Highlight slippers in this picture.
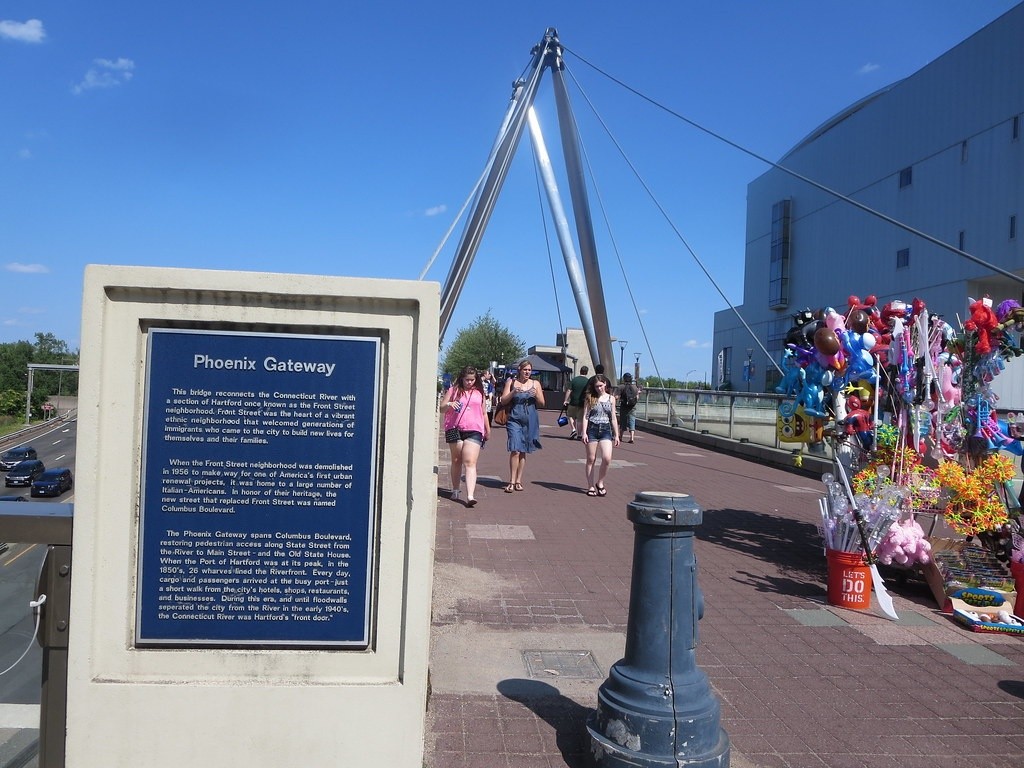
[586,486,597,497]
[596,484,607,496]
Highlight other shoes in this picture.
[450,489,461,500]
[467,497,477,507]
[577,435,582,441]
[619,436,623,442]
[627,441,634,443]
[567,430,577,439]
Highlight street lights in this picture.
[745,348,753,393]
[617,340,629,384]
[686,370,696,390]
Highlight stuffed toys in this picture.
[965,299,1003,354]
[872,519,931,568]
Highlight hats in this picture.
[623,373,633,377]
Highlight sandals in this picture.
[505,482,514,493]
[515,482,524,491]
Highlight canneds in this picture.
[454,401,463,413]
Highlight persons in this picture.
[563,364,611,440]
[615,373,639,443]
[582,375,620,497]
[479,370,517,426]
[1010,452,1024,626]
[440,366,491,506]
[502,361,545,493]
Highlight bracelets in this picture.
[448,402,450,407]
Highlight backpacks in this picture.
[622,382,637,406]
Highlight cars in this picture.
[30,467,72,498]
[6,459,45,488]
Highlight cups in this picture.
[454,402,463,413]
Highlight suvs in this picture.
[1,446,37,472]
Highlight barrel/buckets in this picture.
[824,543,875,610]
[1010,559,1024,619]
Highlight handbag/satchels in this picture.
[494,379,515,426]
[557,405,568,427]
[445,428,461,443]
